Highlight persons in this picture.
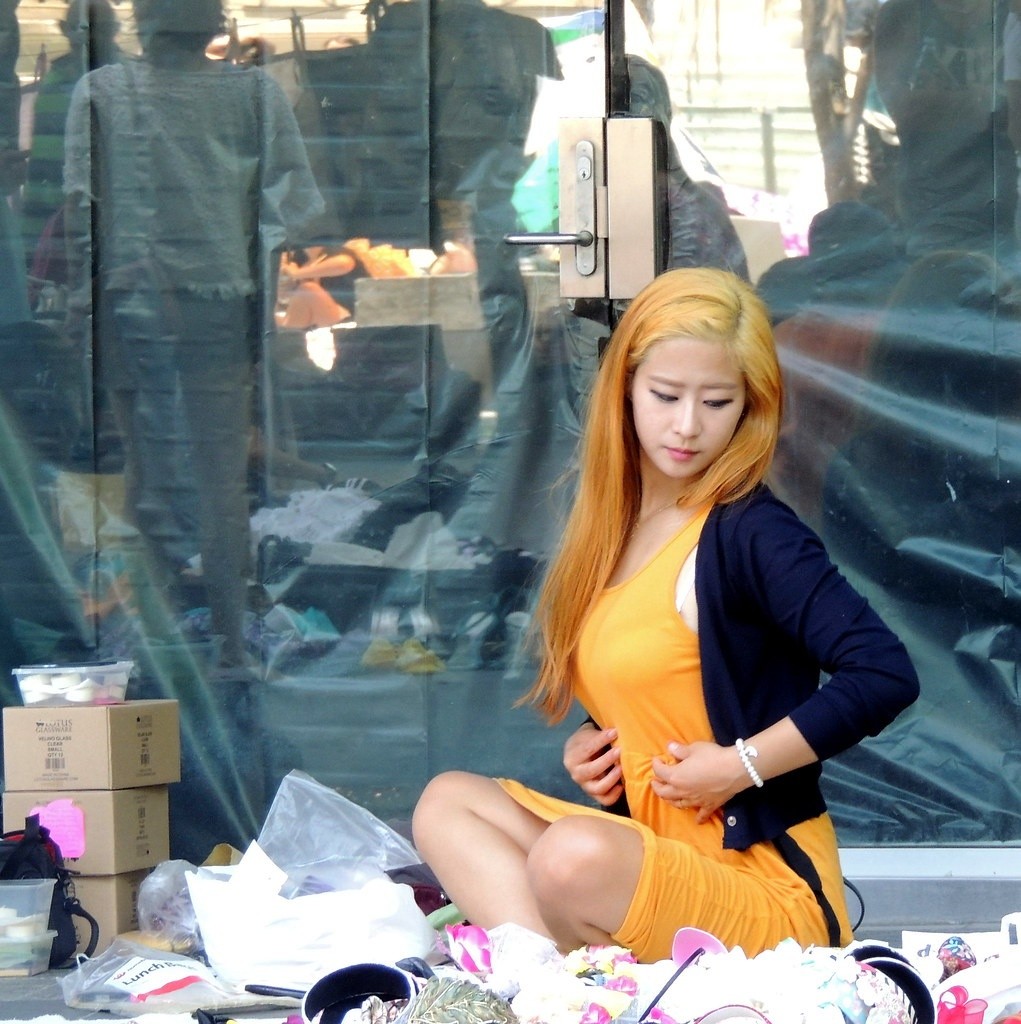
[0,0,1021,845]
[412,265,924,964]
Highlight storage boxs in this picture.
[355,274,574,413]
[0,659,180,978]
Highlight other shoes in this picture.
[504,614,541,673]
[447,614,504,669]
[361,637,399,669]
[397,638,448,675]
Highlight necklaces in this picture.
[622,500,677,543]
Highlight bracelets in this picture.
[734,738,764,788]
